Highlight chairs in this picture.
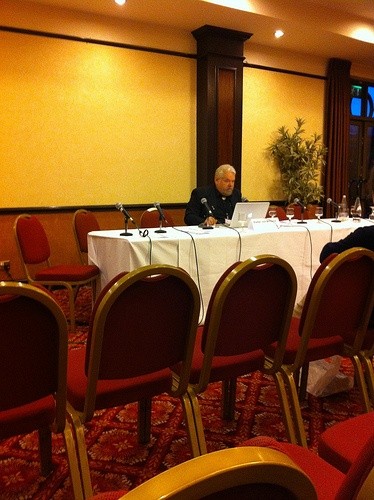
[0,201,374,500]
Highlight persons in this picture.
[296,225,374,311]
[184,164,242,225]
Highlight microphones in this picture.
[294,198,306,209]
[327,198,339,209]
[201,198,213,215]
[115,203,136,225]
[154,201,166,221]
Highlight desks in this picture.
[86,217,374,327]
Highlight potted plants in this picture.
[264,115,328,212]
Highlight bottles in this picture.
[354,197,361,218]
[338,194,349,221]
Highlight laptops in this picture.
[223,202,270,228]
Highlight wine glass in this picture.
[350,206,357,221]
[314,206,324,223]
[368,206,374,221]
[285,207,295,222]
[268,205,278,218]
